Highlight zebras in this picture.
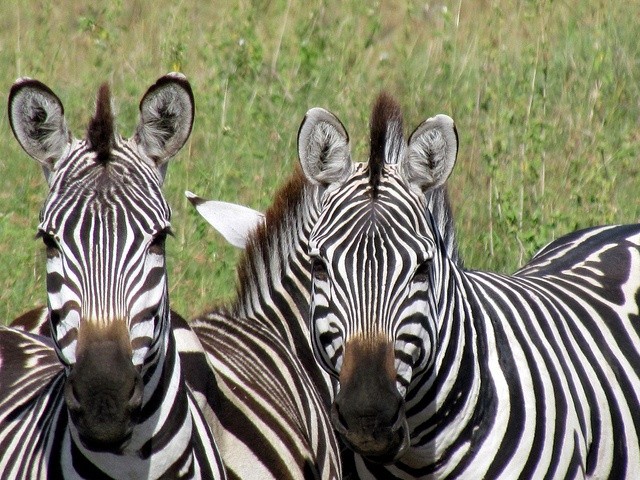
[295,89,639,480]
[183,166,341,480]
[1,72,227,480]
